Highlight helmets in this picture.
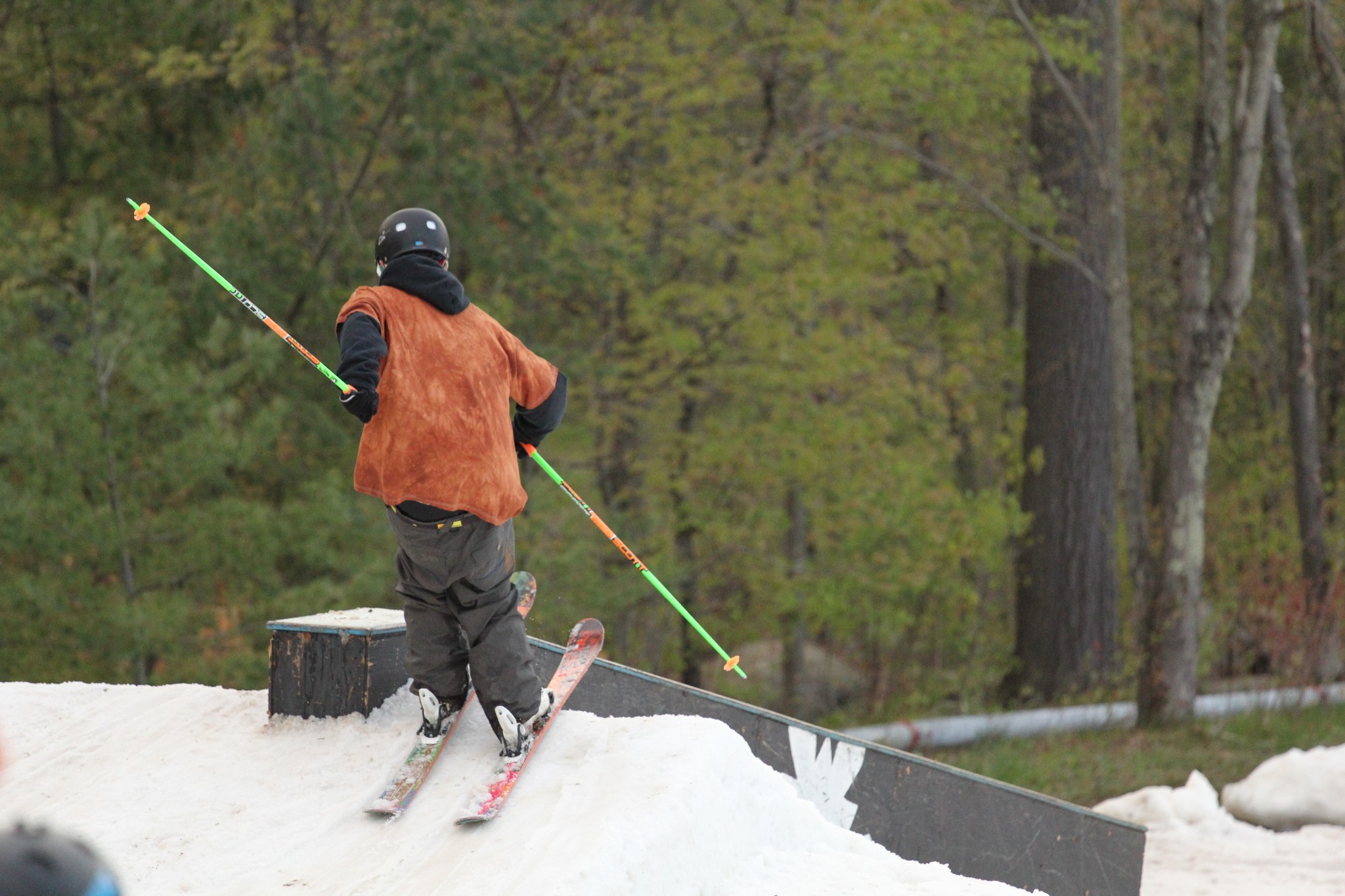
[373,207,448,265]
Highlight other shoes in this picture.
[419,687,460,744]
[493,688,554,771]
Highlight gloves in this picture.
[339,387,379,423]
[511,423,540,460]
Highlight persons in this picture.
[332,207,569,745]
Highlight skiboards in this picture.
[367,570,605,828]
